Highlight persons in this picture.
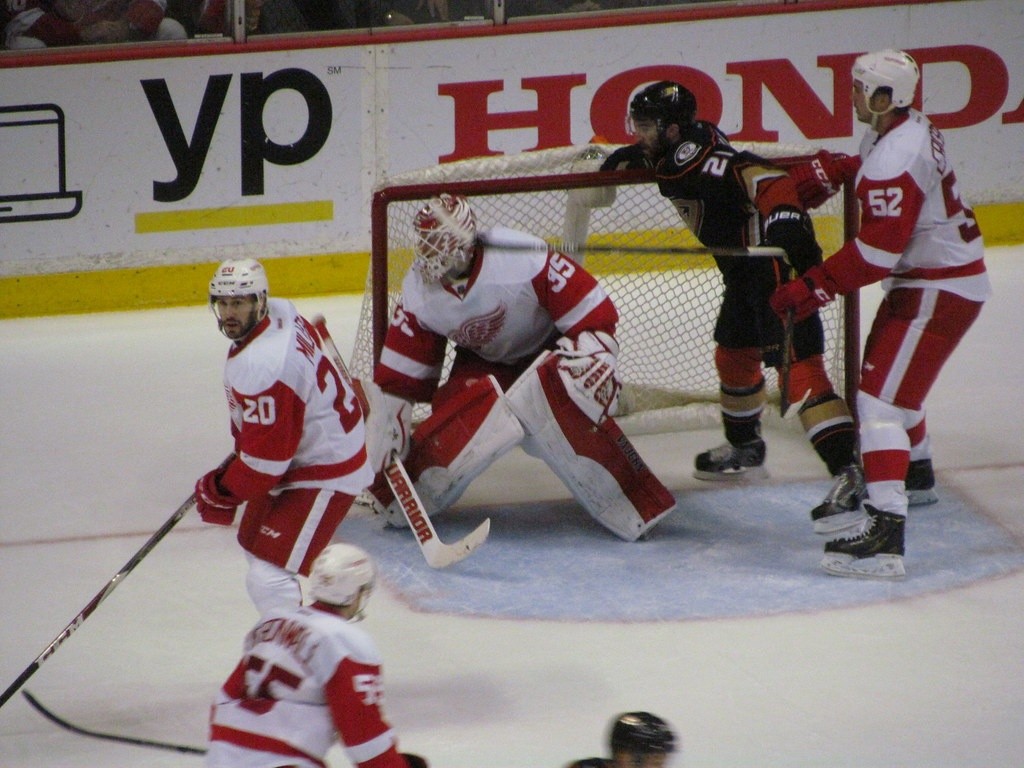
[364,194,680,541]
[565,713,677,768]
[772,49,997,582]
[601,81,871,533]
[193,257,375,617]
[204,543,427,768]
[0,0,719,51]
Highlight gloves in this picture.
[767,265,836,323]
[601,145,653,171]
[790,150,842,208]
[765,205,819,262]
[196,467,242,525]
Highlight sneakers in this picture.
[904,457,939,505]
[694,420,765,480]
[821,504,906,576]
[811,463,870,533]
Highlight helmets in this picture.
[414,194,477,284]
[307,543,373,606]
[628,80,694,140]
[611,712,675,754]
[209,257,268,320]
[854,47,920,114]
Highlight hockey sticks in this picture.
[310,313,491,569]
[21,690,208,754]
[429,196,785,257]
[780,264,813,420]
[0,492,197,708]
